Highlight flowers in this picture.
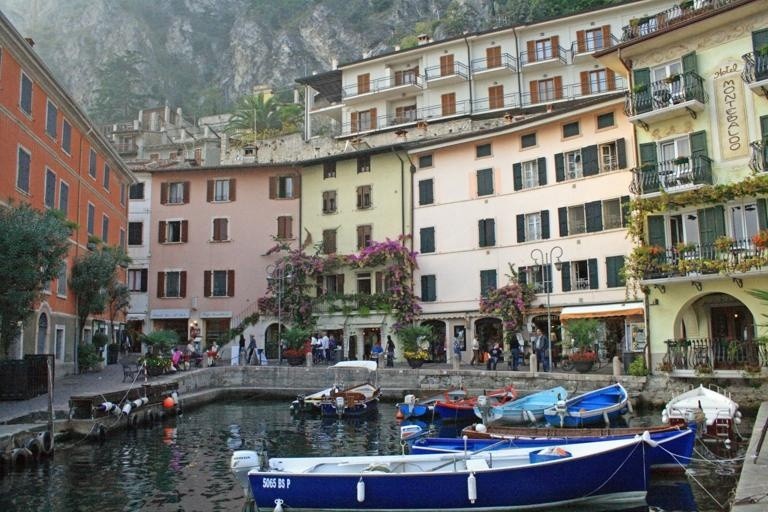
[568,351,596,363]
[283,348,307,360]
[403,349,431,361]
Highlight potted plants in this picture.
[93,356,106,372]
[138,356,166,377]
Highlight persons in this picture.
[171,329,556,374]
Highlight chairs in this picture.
[119,358,138,382]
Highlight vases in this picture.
[575,361,591,373]
[407,357,423,368]
[286,356,304,367]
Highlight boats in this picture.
[645,472,697,510]
[70,377,184,440]
[227,431,653,511]
[290,357,382,419]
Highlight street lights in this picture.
[529,244,566,371]
[265,261,292,366]
